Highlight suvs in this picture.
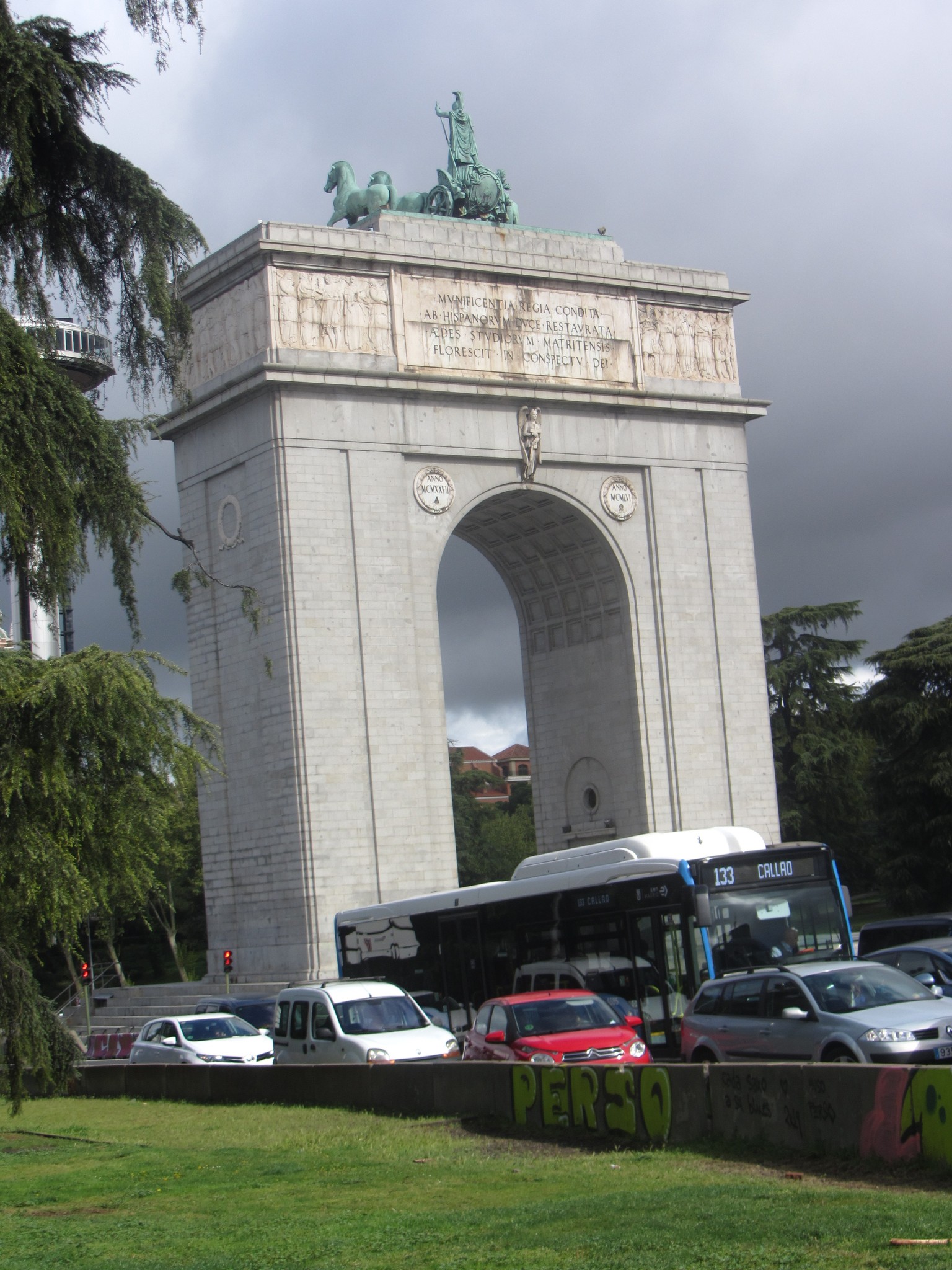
[678,955,952,1065]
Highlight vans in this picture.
[273,974,462,1066]
[511,954,692,1045]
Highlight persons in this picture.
[173,275,268,397]
[767,927,800,964]
[272,267,393,356]
[843,979,866,1008]
[516,402,542,482]
[435,91,484,187]
[638,303,738,384]
[204,1020,227,1040]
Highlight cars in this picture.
[128,1011,274,1065]
[861,935,952,994]
[461,988,654,1065]
[193,993,277,1038]
[406,989,479,1043]
[858,913,952,958]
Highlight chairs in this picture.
[774,982,821,1020]
[550,1004,579,1030]
[360,1006,387,1032]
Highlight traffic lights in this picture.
[224,950,233,972]
[80,961,90,984]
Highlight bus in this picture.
[333,826,857,1064]
